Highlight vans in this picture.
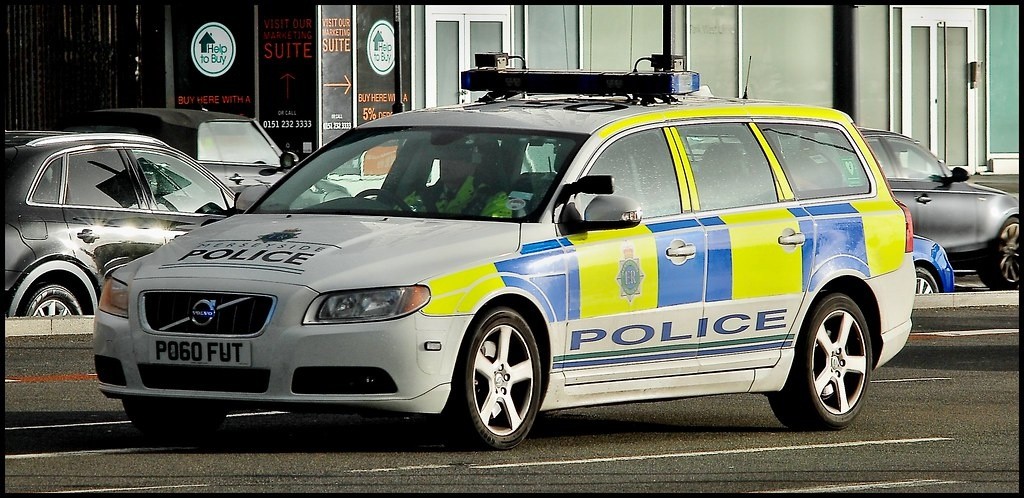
[94,108,289,185]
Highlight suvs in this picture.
[2,131,240,319]
[858,126,1022,292]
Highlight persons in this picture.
[393,139,512,218]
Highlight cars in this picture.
[91,59,915,450]
